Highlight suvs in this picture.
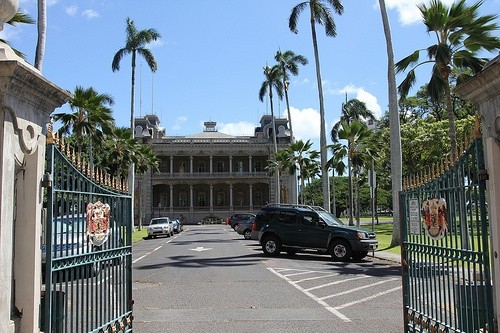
[227,213,255,229]
[41,212,125,277]
[250,203,379,262]
[146,216,174,239]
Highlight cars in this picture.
[236,215,256,240]
[171,220,183,234]
[230,216,253,231]
